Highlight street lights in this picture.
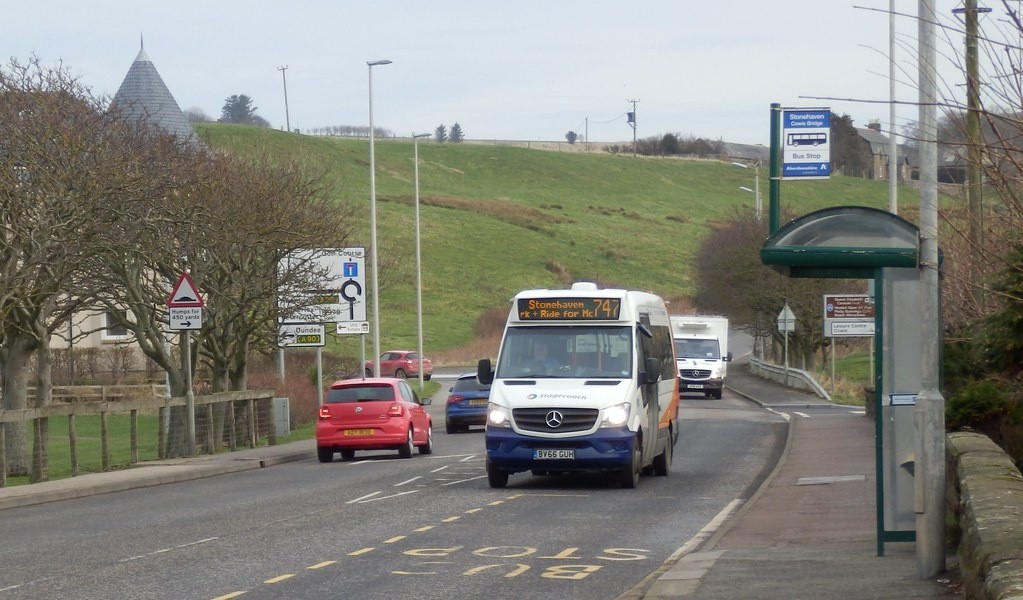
[365,60,396,378]
[410,132,433,391]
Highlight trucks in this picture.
[667,314,732,400]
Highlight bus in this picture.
[787,133,826,147]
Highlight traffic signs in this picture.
[337,321,369,334]
[275,324,325,348]
[169,307,202,330]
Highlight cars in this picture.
[444,371,495,434]
[316,377,433,458]
[365,350,433,382]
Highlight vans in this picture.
[476,282,680,490]
